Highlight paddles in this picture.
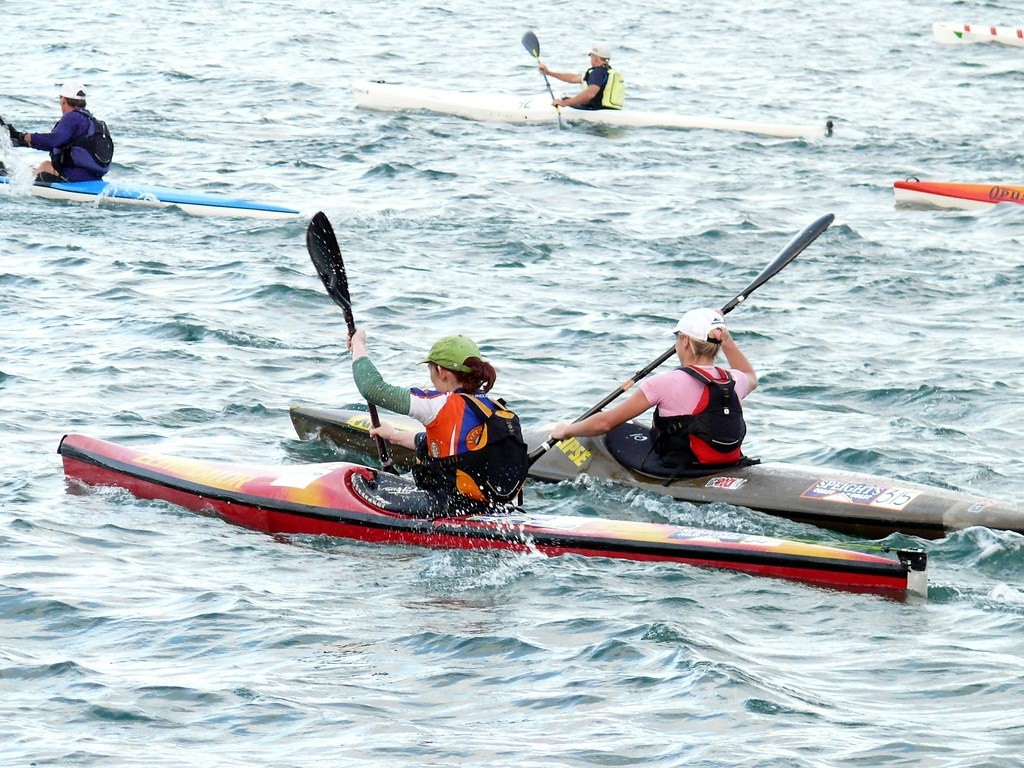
[520,32,572,131]
[304,208,403,474]
[530,213,840,467]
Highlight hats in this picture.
[54,81,87,100]
[663,308,725,344]
[417,335,481,373]
[588,47,610,58]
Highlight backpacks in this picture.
[73,109,113,168]
[597,65,625,110]
[456,393,529,506]
[674,367,747,464]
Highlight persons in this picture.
[538,47,612,110]
[551,308,757,467]
[347,326,495,504]
[4,83,104,181]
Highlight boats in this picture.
[896,175,1024,215]
[0,169,299,221]
[58,431,927,595]
[354,79,862,142]
[289,398,1024,540]
